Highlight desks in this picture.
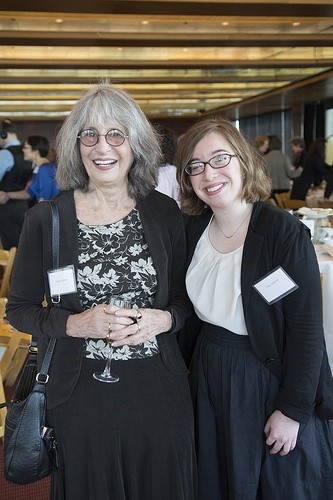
[309,227,333,371]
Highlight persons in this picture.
[251,134,310,212]
[0,120,61,249]
[173,118,333,500]
[153,127,183,209]
[5,78,192,499]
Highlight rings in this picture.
[103,305,106,313]
[106,333,109,340]
[136,323,141,333]
[108,324,110,331]
[136,308,143,321]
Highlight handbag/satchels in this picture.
[3,344,58,485]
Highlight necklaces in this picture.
[214,209,252,240]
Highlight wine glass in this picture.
[93,297,131,382]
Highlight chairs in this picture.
[268,190,333,222]
[0,247,16,299]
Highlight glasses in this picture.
[75,128,130,147]
[182,154,239,176]
[22,146,33,150]
